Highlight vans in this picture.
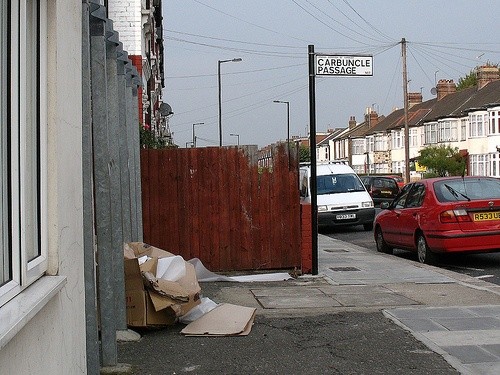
[299,162,375,230]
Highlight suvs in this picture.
[358,175,399,207]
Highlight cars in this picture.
[375,176,500,266]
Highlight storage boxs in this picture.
[121,240,204,330]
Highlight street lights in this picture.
[191,122,206,147]
[217,57,243,149]
[273,100,290,141]
[230,132,240,147]
